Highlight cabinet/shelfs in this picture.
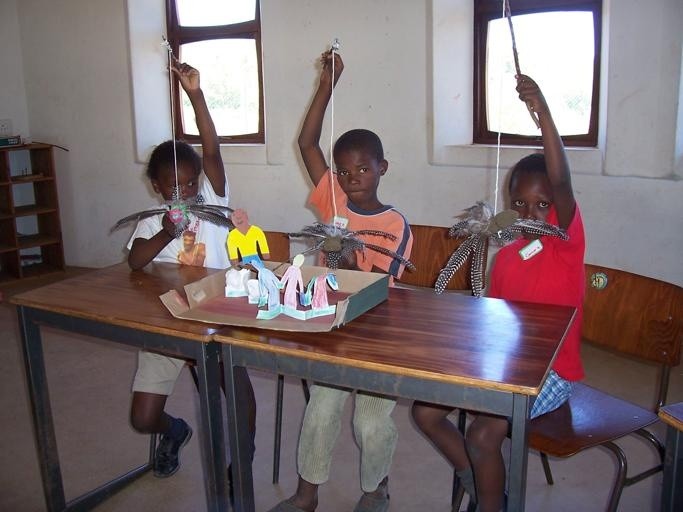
[0,143,67,304]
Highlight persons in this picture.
[115,51,226,478]
[421,69,587,511]
[267,52,415,512]
[175,229,206,267]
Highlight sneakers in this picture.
[153,418,193,479]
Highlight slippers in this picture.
[353,493,390,512]
[268,499,315,512]
